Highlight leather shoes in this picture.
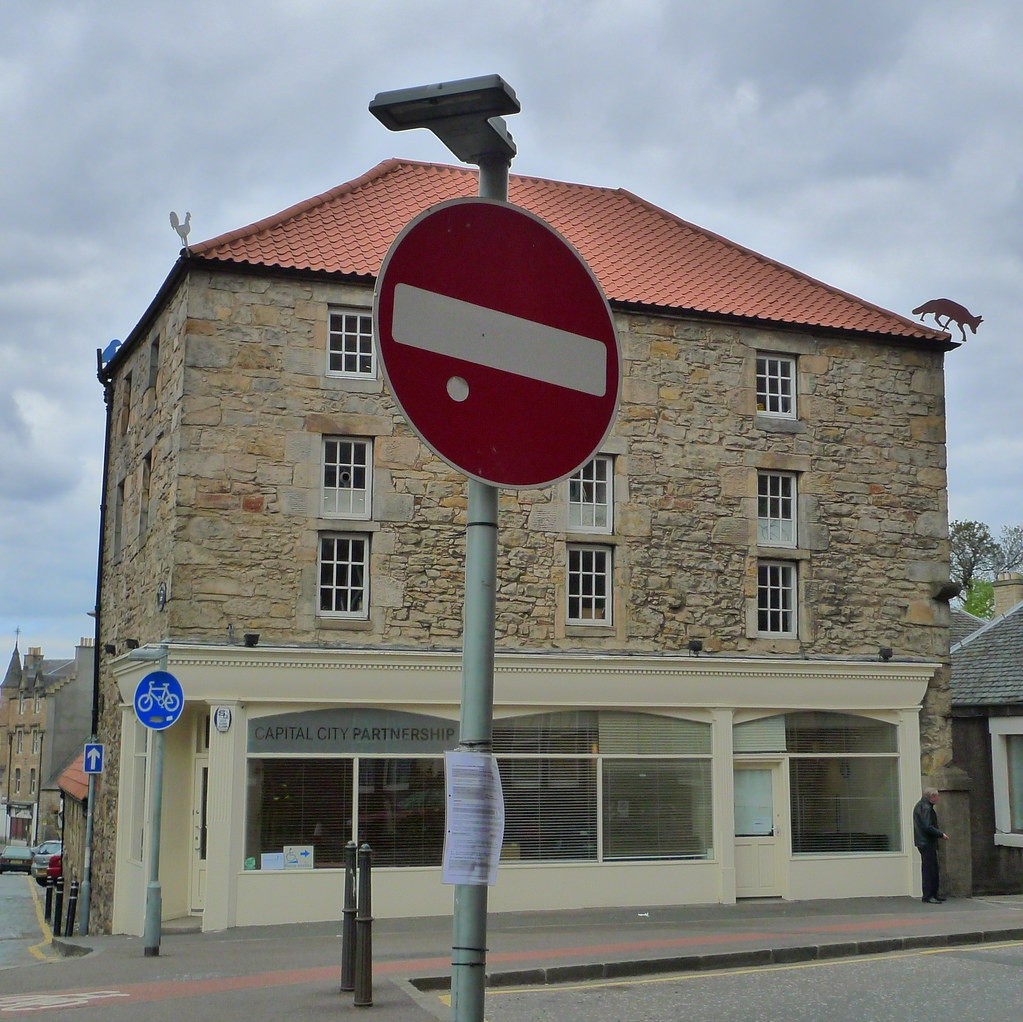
[928,897,941,904]
[935,895,946,901]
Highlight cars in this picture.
[0,846,35,875]
[31,840,63,887]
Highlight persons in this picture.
[912,786,949,903]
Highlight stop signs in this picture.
[370,195,622,494]
